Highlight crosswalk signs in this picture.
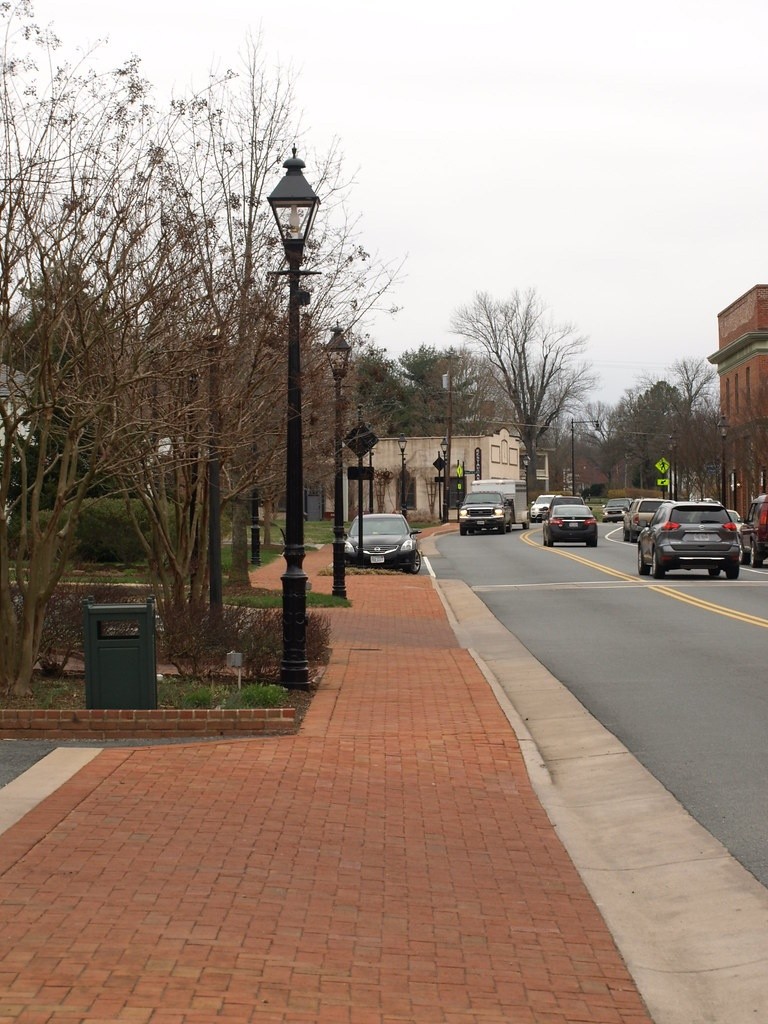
[655,458,670,474]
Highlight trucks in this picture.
[456,479,530,536]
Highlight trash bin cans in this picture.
[81,595,158,710]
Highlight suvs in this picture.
[531,495,593,523]
[737,495,768,568]
[622,498,676,544]
[637,500,744,580]
[602,498,633,523]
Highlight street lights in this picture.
[667,440,673,500]
[267,143,321,692]
[323,319,352,598]
[522,452,530,508]
[398,433,408,518]
[353,405,365,569]
[717,413,730,508]
[671,433,679,501]
[570,418,601,496]
[440,435,449,524]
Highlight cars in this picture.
[344,514,422,575]
[543,505,598,547]
